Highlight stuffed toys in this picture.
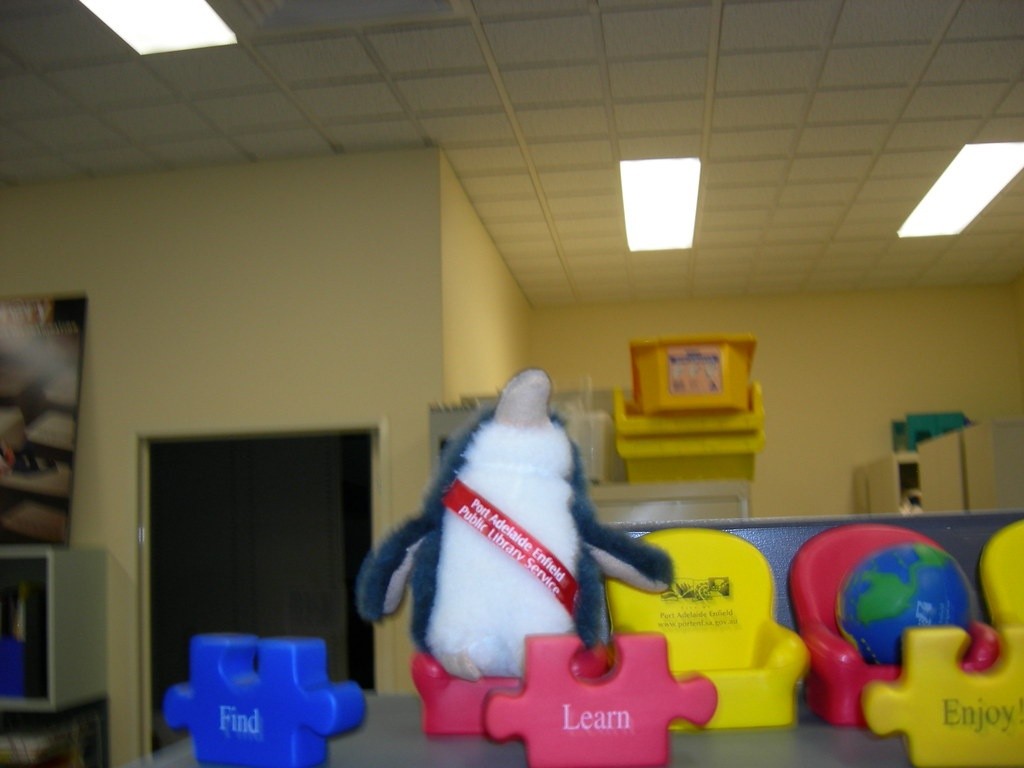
[356,367,673,682]
